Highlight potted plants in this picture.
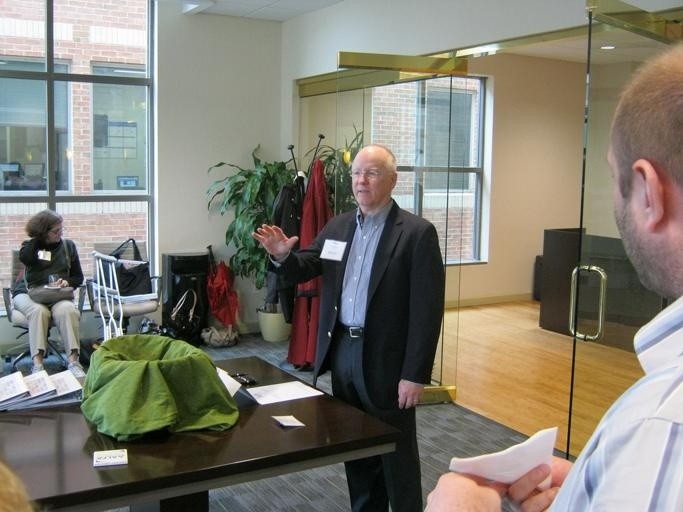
[205,141,307,343]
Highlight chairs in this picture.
[3,238,165,372]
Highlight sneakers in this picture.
[67,361,84,373]
[30,364,44,374]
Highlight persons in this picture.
[419,37,683,512]
[9,208,88,381]
[250,142,446,512]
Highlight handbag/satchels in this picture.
[135,315,167,336]
[28,281,75,304]
[85,236,142,312]
[201,324,239,347]
[78,333,113,365]
[162,288,201,349]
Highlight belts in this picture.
[335,321,363,338]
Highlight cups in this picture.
[48,274,59,287]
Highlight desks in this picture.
[0,354,400,511]
[539,226,672,356]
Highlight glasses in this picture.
[48,226,65,234]
[348,169,378,180]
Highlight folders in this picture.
[19,370,84,410]
[0,371,31,412]
[7,369,57,412]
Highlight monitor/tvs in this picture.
[0,162,21,184]
[116,176,140,187]
